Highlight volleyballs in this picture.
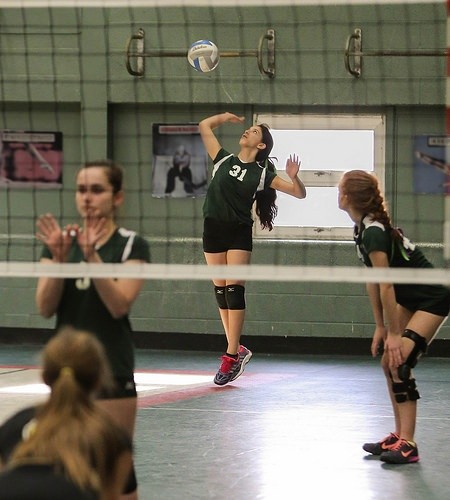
[188,39,220,73]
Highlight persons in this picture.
[34,160,152,500]
[198,111,307,386]
[0,326,134,500]
[338,171,450,464]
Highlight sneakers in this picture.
[363,433,400,455]
[231,345,253,381]
[214,352,239,385]
[380,439,419,463]
